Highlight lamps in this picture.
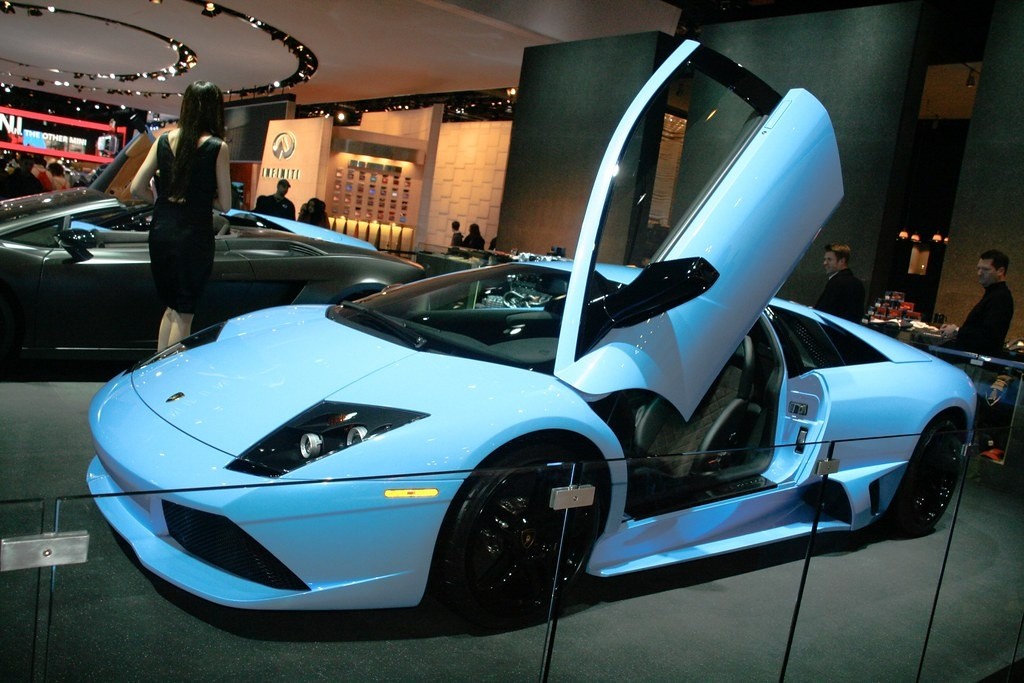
[201,2,222,17]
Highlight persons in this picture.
[940,249,1015,372]
[449,221,485,251]
[253,179,330,229]
[129,80,232,353]
[0,151,70,200]
[815,241,867,324]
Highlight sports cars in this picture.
[82,30,978,636]
[0,126,432,381]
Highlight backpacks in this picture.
[33,165,52,192]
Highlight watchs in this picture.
[953,326,959,336]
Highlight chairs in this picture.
[635,334,756,508]
[213,215,230,237]
